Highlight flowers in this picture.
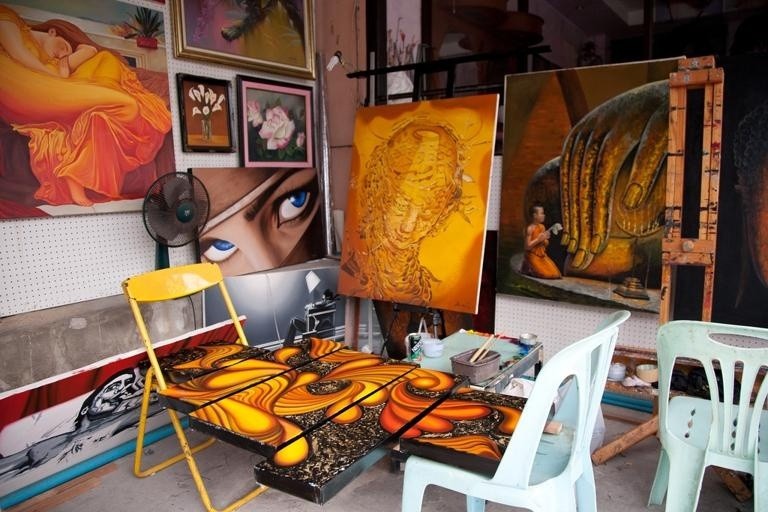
[246,93,308,161]
[185,82,226,120]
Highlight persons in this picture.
[559,78,670,273]
[282,289,334,347]
[341,117,477,306]
[0,368,169,476]
[519,204,562,279]
[1,6,139,207]
[193,167,323,278]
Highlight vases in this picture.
[199,118,213,143]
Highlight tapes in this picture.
[636,364,658,382]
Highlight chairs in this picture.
[645,318,768,511]
[302,304,337,342]
[119,261,271,512]
[398,309,634,512]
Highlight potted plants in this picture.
[121,6,167,53]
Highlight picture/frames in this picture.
[170,0,319,84]
[173,69,239,158]
[233,73,317,169]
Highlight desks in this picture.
[401,327,544,396]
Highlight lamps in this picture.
[304,270,322,294]
[327,52,354,75]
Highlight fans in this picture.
[138,168,213,300]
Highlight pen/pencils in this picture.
[469,334,501,364]
